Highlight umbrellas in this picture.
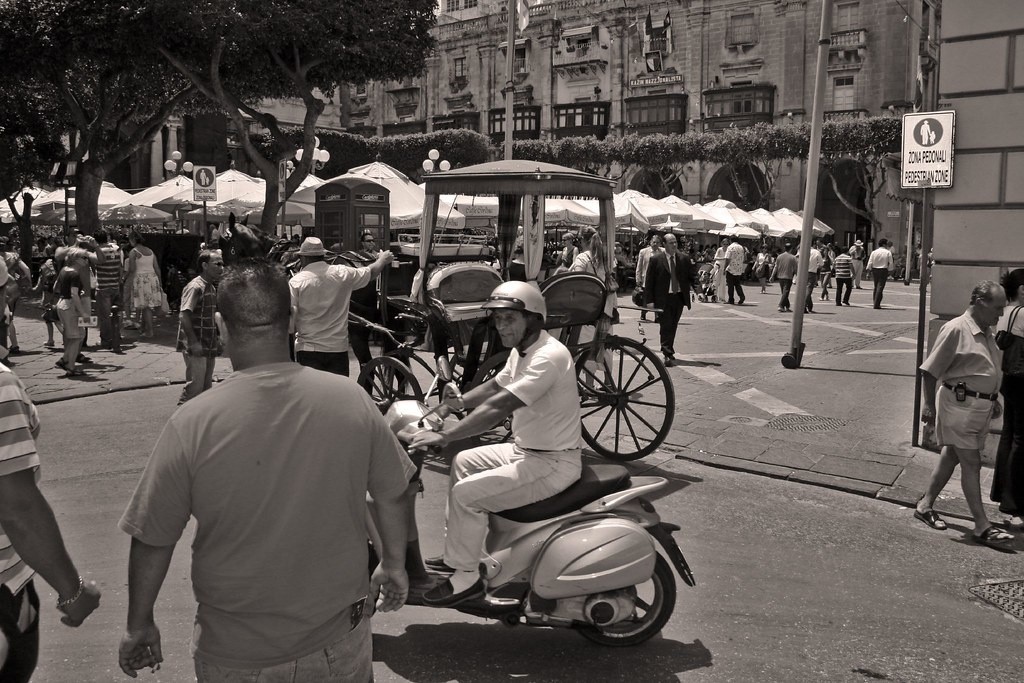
[0,156,844,258]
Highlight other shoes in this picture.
[425,555,456,572]
[1,326,158,377]
[422,573,484,607]
[664,353,674,366]
[717,289,881,314]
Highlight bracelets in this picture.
[56,576,84,607]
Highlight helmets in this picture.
[481,281,546,327]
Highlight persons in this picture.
[912,279,1016,543]
[989,269,1024,517]
[558,233,581,268]
[833,247,856,305]
[695,237,751,304]
[866,239,894,309]
[634,236,665,323]
[0,222,397,403]
[570,225,620,401]
[805,242,825,314]
[116,255,420,683]
[888,241,896,254]
[0,251,104,683]
[406,279,584,608]
[644,232,704,360]
[771,244,798,312]
[849,239,866,289]
[752,238,836,301]
[509,234,528,284]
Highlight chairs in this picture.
[427,262,506,322]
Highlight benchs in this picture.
[539,273,619,329]
[398,234,494,256]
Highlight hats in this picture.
[854,240,864,246]
[294,236,327,256]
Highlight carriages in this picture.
[217,160,675,463]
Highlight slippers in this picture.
[971,525,1014,544]
[913,509,949,530]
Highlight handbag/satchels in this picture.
[632,286,645,306]
[605,274,619,293]
[995,305,1024,374]
[156,288,169,312]
[48,274,57,293]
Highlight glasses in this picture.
[364,239,375,242]
[208,261,226,268]
[80,256,90,260]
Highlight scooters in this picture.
[366,354,696,650]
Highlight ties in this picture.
[670,256,678,295]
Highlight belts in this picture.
[522,447,579,453]
[943,381,999,402]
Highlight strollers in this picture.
[691,263,721,303]
